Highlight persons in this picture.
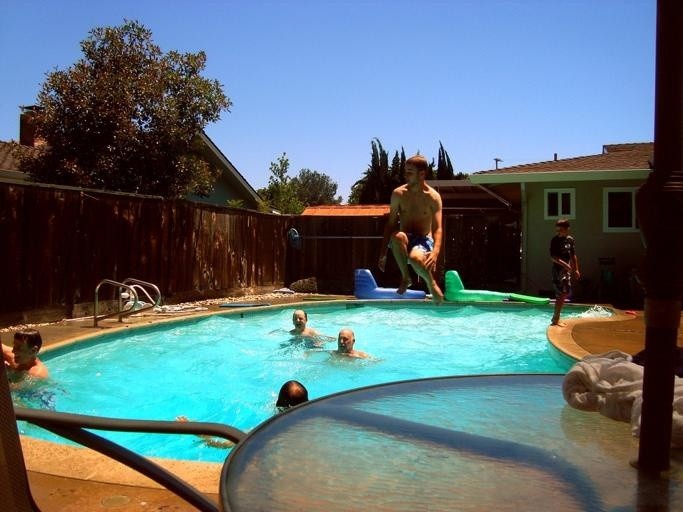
[177,380,308,450]
[549,217,582,327]
[323,329,376,363]
[1,328,57,416]
[376,155,449,302]
[289,306,321,338]
